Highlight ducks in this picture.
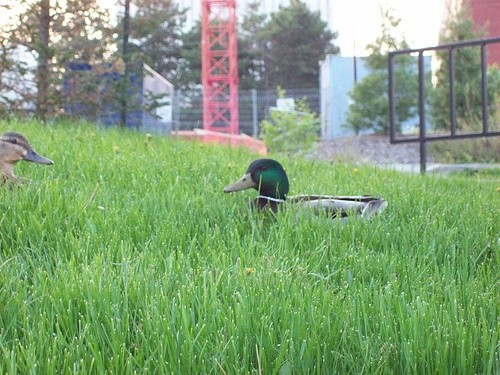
[224,158,388,220]
[0,130,53,188]
